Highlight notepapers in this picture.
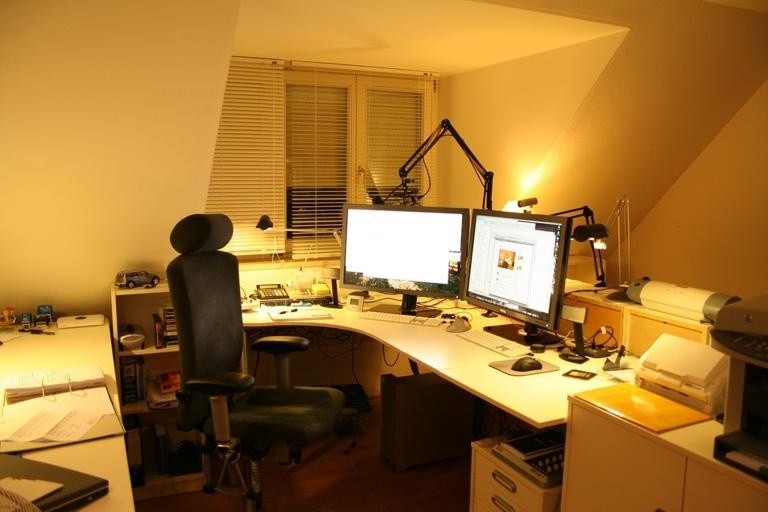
[311,283,330,295]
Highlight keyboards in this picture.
[457,329,531,359]
[359,311,443,327]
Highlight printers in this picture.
[706,290,767,485]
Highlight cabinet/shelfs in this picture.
[560,386,768,512]
[108,280,230,500]
[464,434,563,511]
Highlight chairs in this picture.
[166,213,359,507]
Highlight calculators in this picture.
[256,284,289,298]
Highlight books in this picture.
[254,303,332,321]
[0,363,126,455]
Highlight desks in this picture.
[241,281,640,512]
[0,312,137,512]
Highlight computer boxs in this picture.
[380,373,478,472]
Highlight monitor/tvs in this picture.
[463,208,573,347]
[338,202,470,310]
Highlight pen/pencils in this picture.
[19,329,55,335]
[280,309,297,314]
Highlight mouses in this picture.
[511,357,542,371]
